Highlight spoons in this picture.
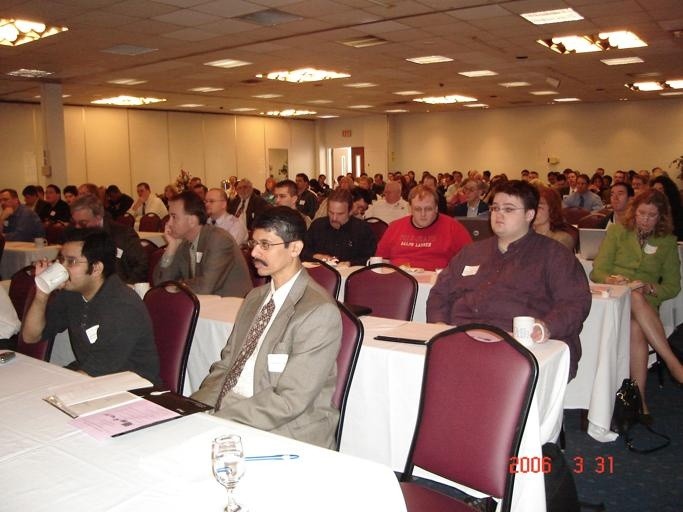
[244,452,303,463]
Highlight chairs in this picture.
[115,211,169,287]
[299,257,342,300]
[143,282,200,395]
[241,243,269,286]
[45,218,89,244]
[330,301,365,452]
[397,320,539,512]
[363,215,387,242]
[7,264,55,362]
[344,263,418,321]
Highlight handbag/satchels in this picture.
[611,378,641,435]
[543,443,578,511]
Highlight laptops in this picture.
[579,229,607,260]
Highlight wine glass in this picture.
[210,434,244,512]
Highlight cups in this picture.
[33,238,47,249]
[511,315,544,350]
[365,256,382,272]
[33,262,70,294]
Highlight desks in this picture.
[49,282,571,511]
[317,254,632,444]
[0,241,62,280]
[1,346,408,511]
[576,239,682,340]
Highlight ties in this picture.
[235,199,247,218]
[214,295,274,414]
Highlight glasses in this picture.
[248,240,293,249]
[489,204,525,214]
[55,256,88,267]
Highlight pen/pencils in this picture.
[245,454,300,460]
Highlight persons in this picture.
[2,164,683,452]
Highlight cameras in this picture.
[0,352,15,363]
